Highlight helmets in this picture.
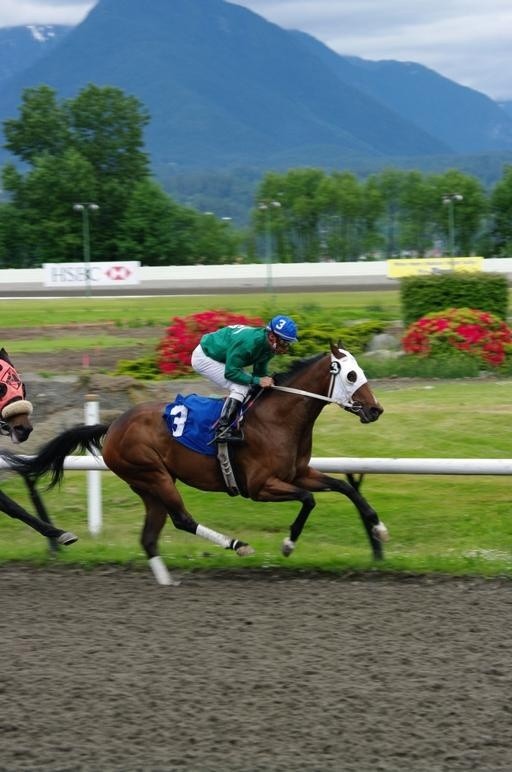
[266,314,297,341]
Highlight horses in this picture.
[1,343,79,547]
[14,336,392,588]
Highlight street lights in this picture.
[442,192,464,255]
[71,201,100,295]
[258,200,281,298]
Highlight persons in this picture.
[188,313,301,442]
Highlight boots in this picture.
[215,397,243,442]
